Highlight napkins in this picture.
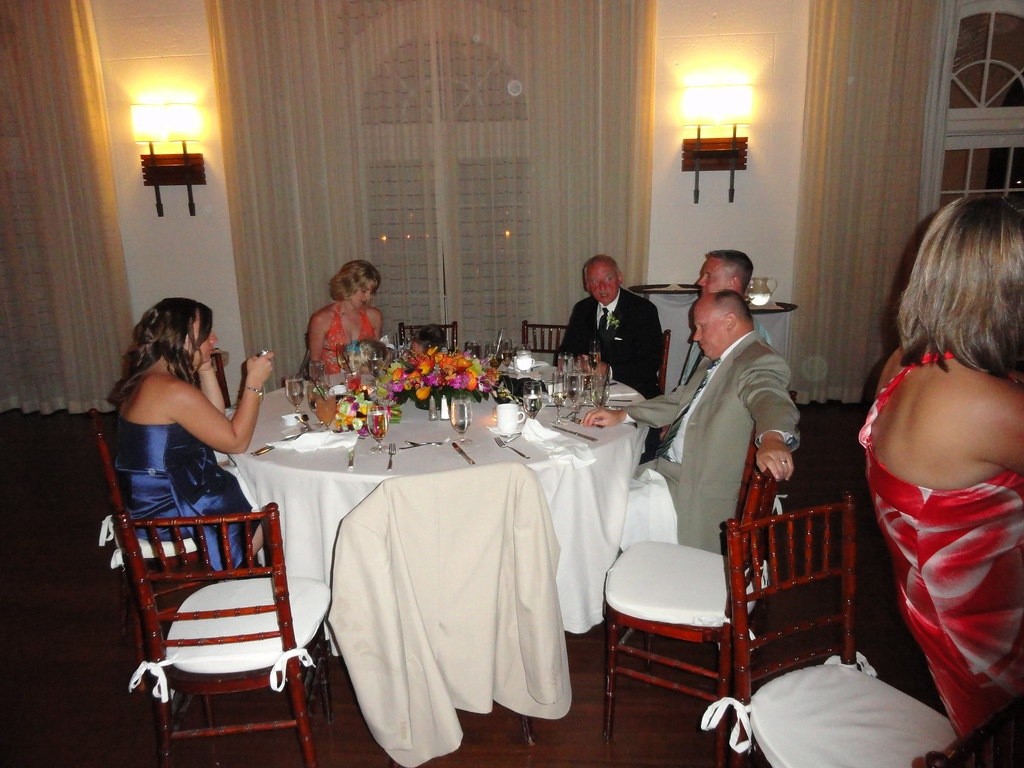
[270,429,359,452]
[521,418,562,445]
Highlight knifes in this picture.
[553,425,598,442]
[452,443,474,465]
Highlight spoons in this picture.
[405,440,441,445]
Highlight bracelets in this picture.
[245,385,262,396]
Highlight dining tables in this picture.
[216,356,648,768]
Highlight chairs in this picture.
[602,457,780,768]
[397,320,672,399]
[726,489,970,768]
[91,408,221,691]
[735,391,797,526]
[173,346,233,410]
[117,502,335,768]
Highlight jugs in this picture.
[745,277,777,306]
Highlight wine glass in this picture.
[285,339,610,454]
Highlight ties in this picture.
[655,359,721,459]
[598,307,609,347]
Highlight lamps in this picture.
[134,91,208,217]
[679,68,757,202]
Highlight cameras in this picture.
[493,375,548,403]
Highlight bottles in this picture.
[441,395,450,420]
[429,395,438,420]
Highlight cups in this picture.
[495,404,525,434]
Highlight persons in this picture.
[587,289,801,555]
[859,195,1024,736]
[308,260,392,375]
[407,324,446,355]
[111,298,275,571]
[554,254,664,400]
[681,249,771,386]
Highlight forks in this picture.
[493,437,530,459]
[399,437,450,450]
[387,443,396,469]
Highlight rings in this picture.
[781,461,787,464]
